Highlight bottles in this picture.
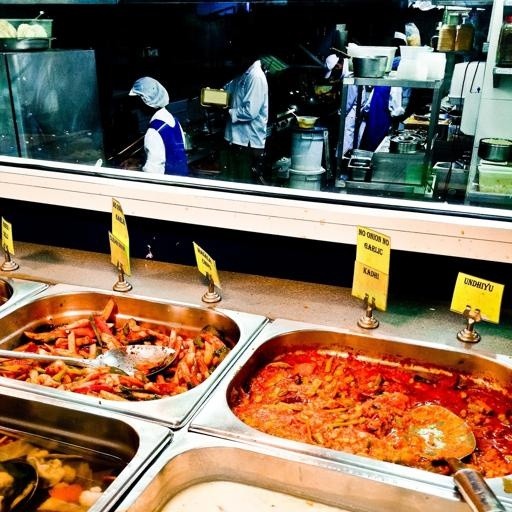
[495,14,512,68]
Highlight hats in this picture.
[322,54,340,79]
[126,75,171,109]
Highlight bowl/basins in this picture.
[296,115,321,128]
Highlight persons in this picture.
[320,52,356,83]
[205,35,271,186]
[336,68,366,173]
[120,74,189,181]
[354,56,412,156]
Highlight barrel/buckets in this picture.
[292,132,323,171]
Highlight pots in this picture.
[478,138,512,162]
[388,132,427,154]
[330,47,388,78]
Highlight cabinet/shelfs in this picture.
[335,72,443,196]
[465,1,512,209]
